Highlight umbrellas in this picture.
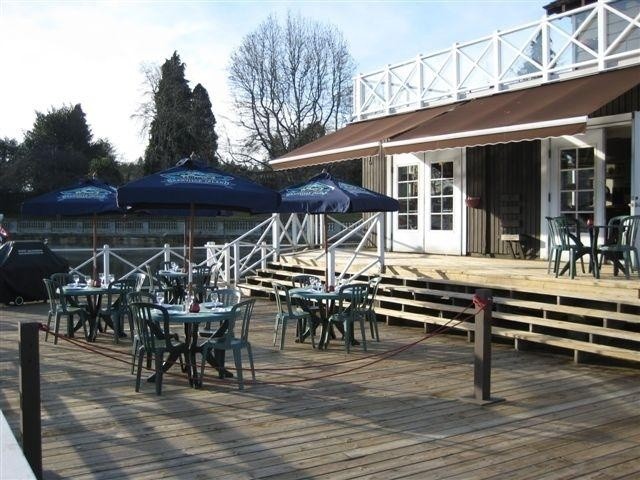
[118,152,281,342]
[19,176,124,283]
[278,167,399,317]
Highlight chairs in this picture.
[545,215,639,280]
[273,275,382,354]
[42,273,146,344]
[156,261,217,305]
[125,290,258,395]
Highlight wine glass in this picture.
[314,279,322,293]
[156,292,167,306]
[108,274,114,286]
[171,261,177,271]
[99,273,104,285]
[309,276,315,291]
[210,293,218,310]
[84,275,91,288]
[73,274,80,286]
